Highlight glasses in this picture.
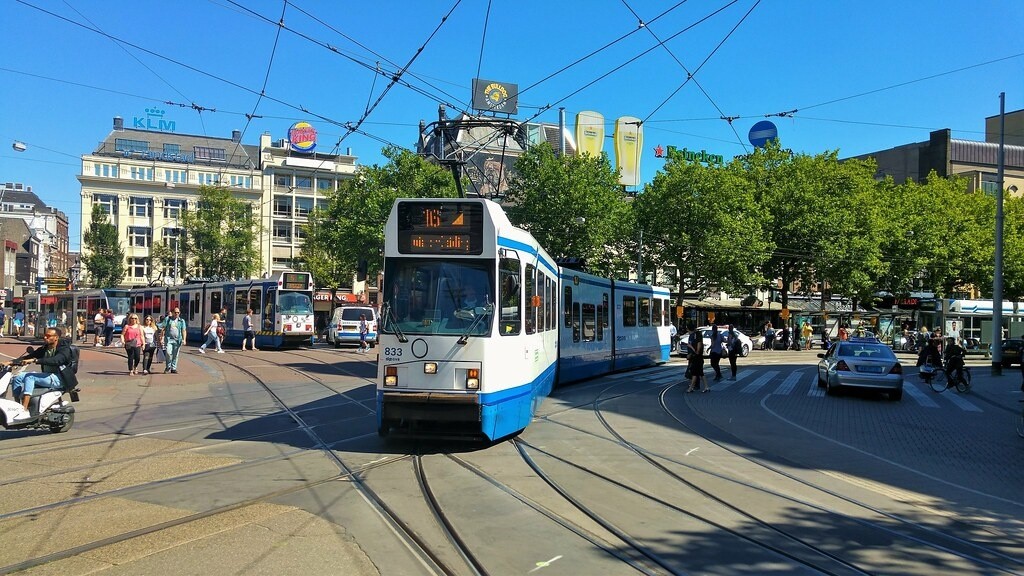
[131,312,180,321]
[45,334,57,338]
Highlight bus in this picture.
[22,288,132,337]
[11,296,26,336]
[919,298,1024,350]
[128,270,318,349]
[375,105,682,441]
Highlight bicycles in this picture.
[929,355,972,394]
[1014,396,1024,439]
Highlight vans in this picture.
[324,306,379,349]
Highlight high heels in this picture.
[702,388,710,393]
[687,387,695,392]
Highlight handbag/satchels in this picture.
[126,338,137,349]
[106,318,115,327]
[157,347,165,361]
[720,337,742,357]
[685,367,692,380]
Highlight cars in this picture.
[675,327,753,359]
[1002,337,1024,366]
[817,335,904,401]
[750,327,792,351]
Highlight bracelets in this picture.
[33,358,37,362]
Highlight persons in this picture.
[670,320,968,395]
[0,301,329,420]
[948,322,961,347]
[446,280,488,330]
[355,314,371,353]
[624,311,657,326]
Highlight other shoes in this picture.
[714,374,722,380]
[356,347,362,351]
[171,370,179,373]
[964,384,971,393]
[365,347,370,352]
[252,348,259,351]
[144,370,148,374]
[14,410,31,419]
[198,348,205,353]
[164,368,171,374]
[217,350,225,353]
[129,368,138,375]
[242,349,247,351]
[0,334,3,338]
[93,343,110,347]
[727,377,736,380]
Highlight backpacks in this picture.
[205,324,212,332]
[68,345,79,373]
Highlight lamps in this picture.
[163,182,175,190]
[12,142,26,152]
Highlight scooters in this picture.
[0,346,82,435]
[918,364,938,382]
